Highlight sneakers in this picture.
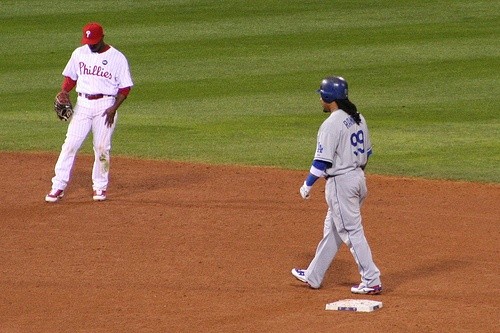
[92,189,107,201]
[291,268,320,289]
[351,284,382,295]
[45,188,65,202]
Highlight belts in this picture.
[79,92,111,100]
[325,174,335,180]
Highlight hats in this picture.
[81,22,103,44]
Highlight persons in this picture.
[290,75,383,295]
[46,23,134,203]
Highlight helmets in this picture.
[317,76,348,103]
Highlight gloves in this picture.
[300,181,312,199]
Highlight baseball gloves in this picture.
[53,91,74,121]
